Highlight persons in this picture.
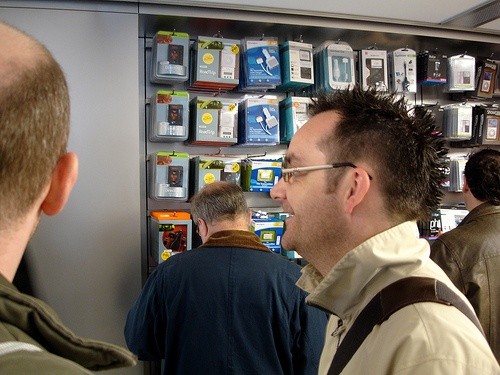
[123,181,328,375]
[431,149,500,363]
[269,83,500,375]
[0,22,137,375]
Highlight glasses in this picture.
[279,158,373,182]
